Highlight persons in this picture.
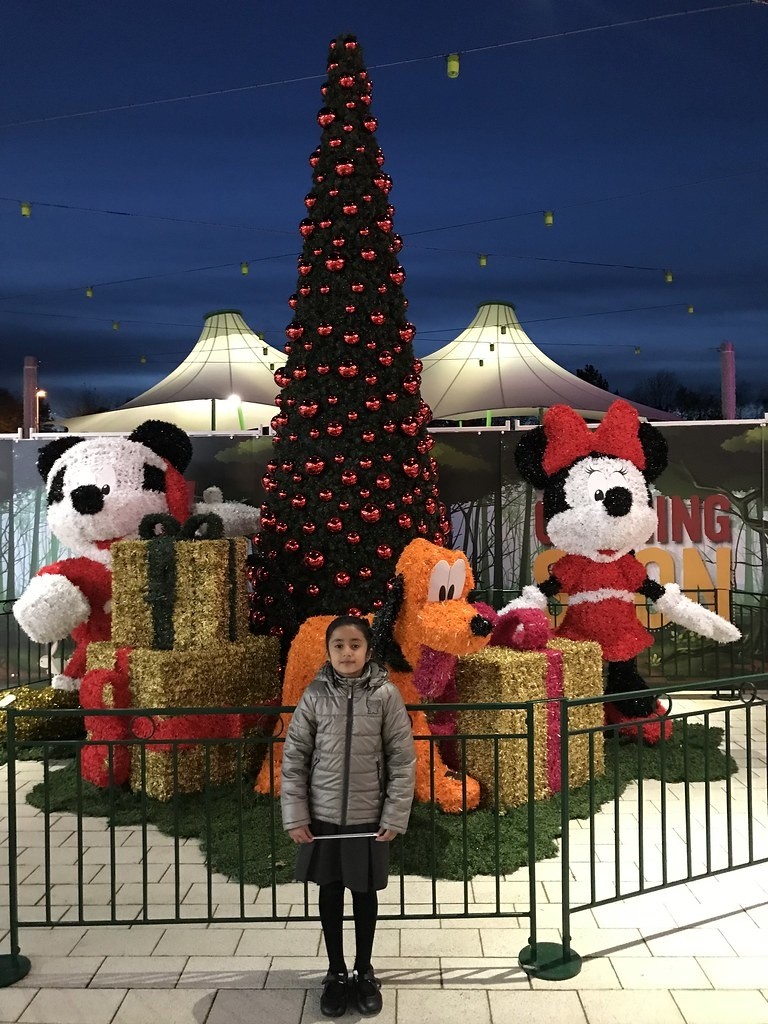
[279,616,415,1017]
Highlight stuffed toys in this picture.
[11,420,261,691]
[496,399,742,741]
[254,538,494,812]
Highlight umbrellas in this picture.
[419,303,680,426]
[47,309,288,432]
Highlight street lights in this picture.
[36,389,47,437]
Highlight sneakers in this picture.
[351,965,383,1015]
[320,964,350,1018]
